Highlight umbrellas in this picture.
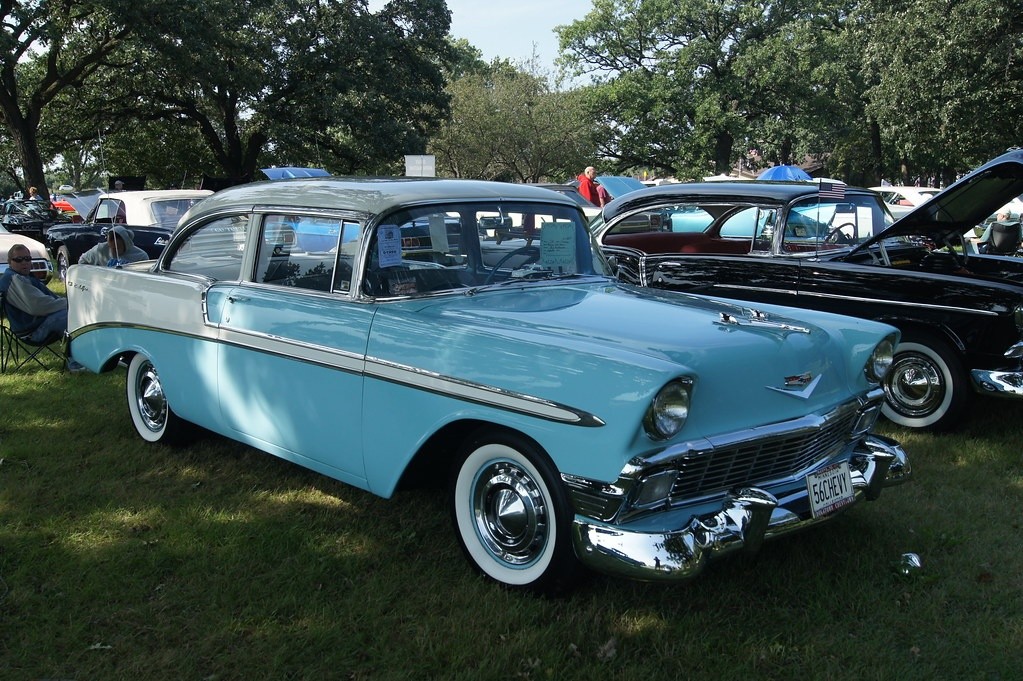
[757,163,816,182]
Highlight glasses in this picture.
[9,255,32,263]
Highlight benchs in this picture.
[274,268,478,306]
[172,256,354,289]
[603,230,720,267]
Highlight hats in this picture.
[115,181,124,185]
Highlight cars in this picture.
[47,188,249,280]
[446,183,601,237]
[0,200,61,243]
[260,167,461,253]
[481,149,1023,430]
[51,198,77,213]
[59,185,73,190]
[14,191,43,201]
[67,176,912,598]
[868,186,942,218]
[0,224,54,286]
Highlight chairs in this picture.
[0,296,71,372]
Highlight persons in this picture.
[49,192,66,202]
[576,164,602,208]
[115,180,125,191]
[79,225,149,268]
[596,183,610,208]
[28,186,43,201]
[1,194,15,203]
[1,242,88,371]
